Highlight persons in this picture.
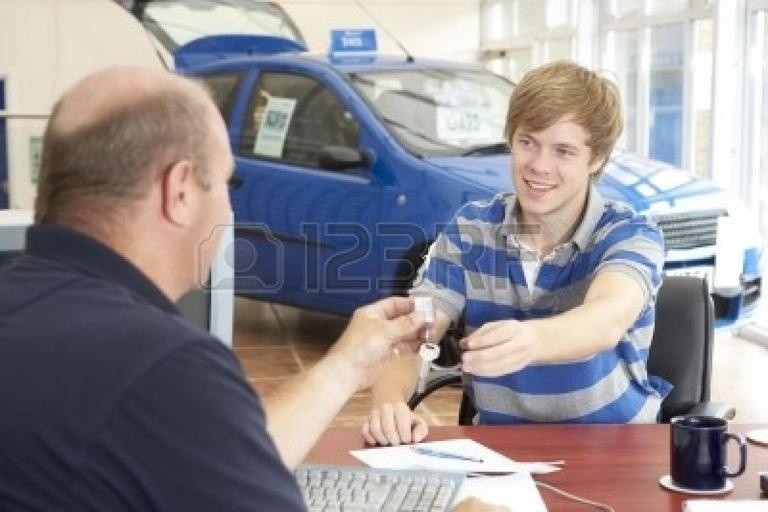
[362,59,674,447]
[0,64,511,512]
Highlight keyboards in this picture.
[285,467,464,512]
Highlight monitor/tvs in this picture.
[0,209,235,351]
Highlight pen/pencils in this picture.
[413,448,483,463]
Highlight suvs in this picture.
[113,1,762,370]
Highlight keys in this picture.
[417,342,441,395]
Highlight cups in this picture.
[670,414,748,491]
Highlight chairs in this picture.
[405,275,738,425]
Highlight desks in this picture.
[305,424,768,512]
